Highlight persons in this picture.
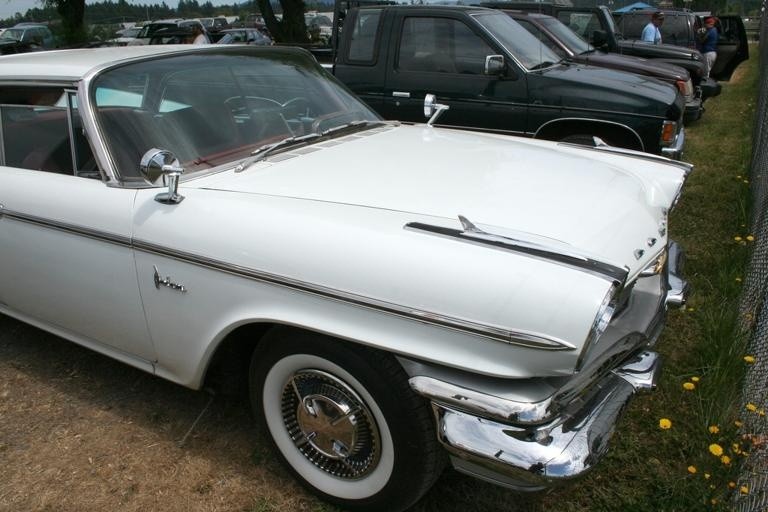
[190,22,207,44]
[309,24,328,47]
[641,11,664,44]
[696,18,719,79]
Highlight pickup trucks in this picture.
[296,2,687,165]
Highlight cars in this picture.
[1,24,55,55]
[106,10,338,46]
[488,0,760,127]
[0,40,696,510]
